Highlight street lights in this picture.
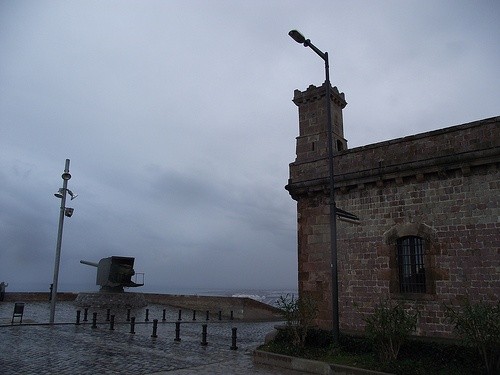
[49,158,79,324]
[289,30,359,347]
